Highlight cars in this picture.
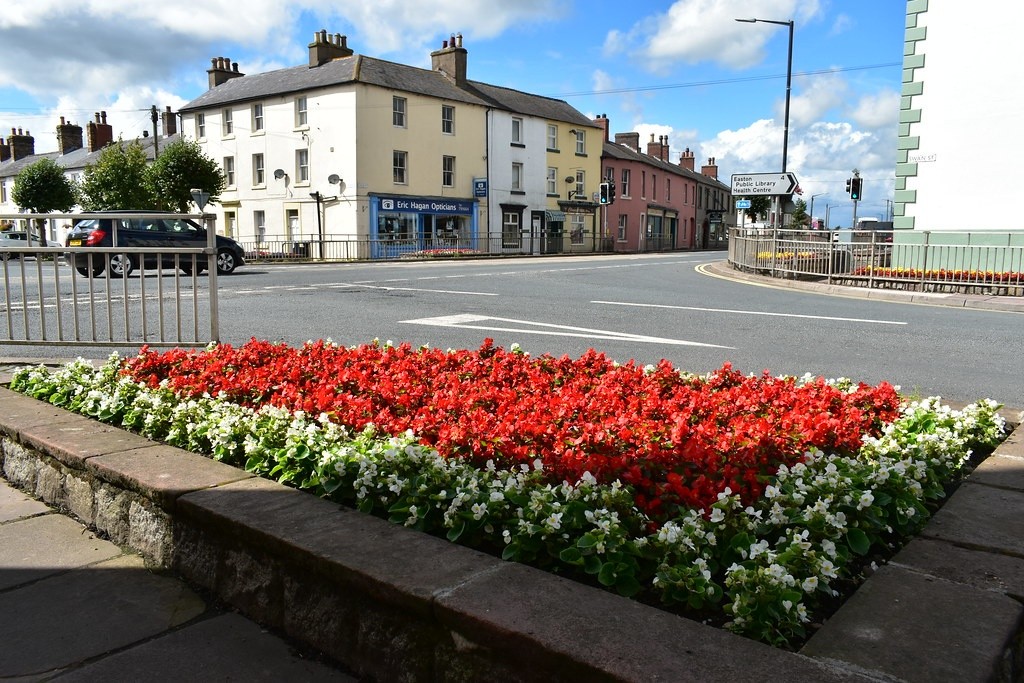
[0,231,63,261]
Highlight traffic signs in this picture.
[730,172,798,196]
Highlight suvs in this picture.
[63,207,245,279]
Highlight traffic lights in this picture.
[599,183,610,205]
[846,178,851,194]
[850,178,861,201]
[609,182,616,204]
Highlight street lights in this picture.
[733,17,795,172]
